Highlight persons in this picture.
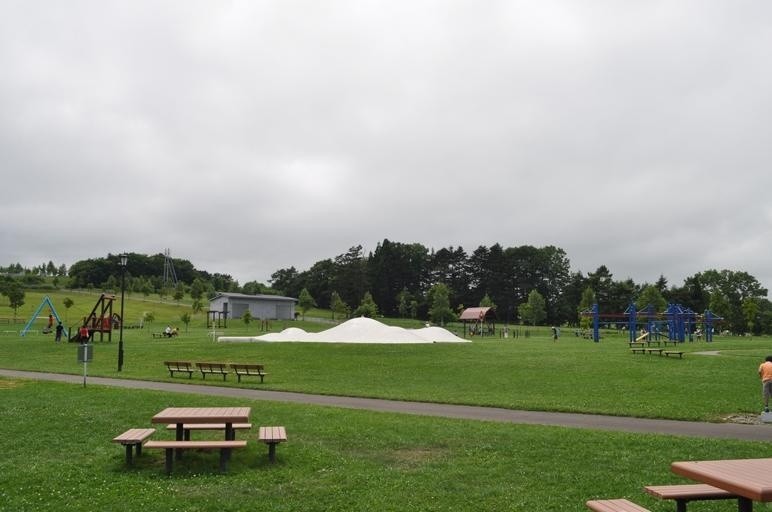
[166,325,172,338]
[171,326,180,338]
[47,314,55,328]
[640,327,646,342]
[79,325,90,344]
[54,322,63,341]
[92,311,98,328]
[757,354,772,412]
[552,326,560,342]
[695,326,702,343]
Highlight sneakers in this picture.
[765,407,769,412]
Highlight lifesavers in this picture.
[479,310,484,322]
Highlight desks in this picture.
[670,458,771,512]
[153,406,252,459]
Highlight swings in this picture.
[42,301,54,333]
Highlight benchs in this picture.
[642,484,743,512]
[163,361,268,383]
[111,429,156,466]
[166,422,253,441]
[259,424,287,462]
[586,497,651,512]
[145,440,247,475]
[628,341,686,358]
